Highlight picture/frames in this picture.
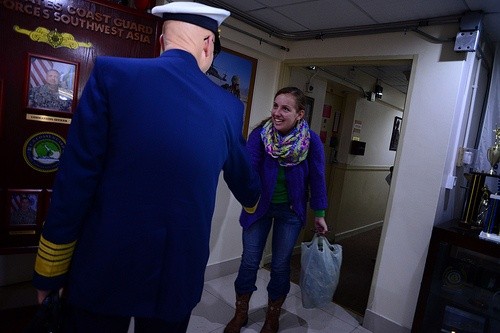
[6,189,52,232]
[206,46,258,141]
[22,53,79,117]
[390,117,402,151]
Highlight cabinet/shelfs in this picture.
[411,227,500,333]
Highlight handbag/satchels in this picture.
[300,233,342,308]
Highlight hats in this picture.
[152,2,231,54]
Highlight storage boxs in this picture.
[443,306,486,333]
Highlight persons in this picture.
[224,87,328,332]
[34,1,262,333]
[29,69,71,113]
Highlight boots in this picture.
[224,291,253,333]
[260,295,286,333]
